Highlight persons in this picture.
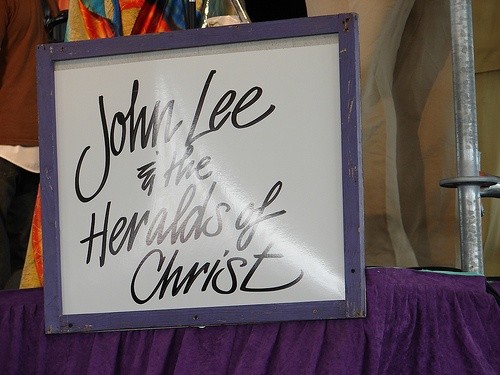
[0,0,57,294]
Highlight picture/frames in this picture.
[35,12,367,336]
